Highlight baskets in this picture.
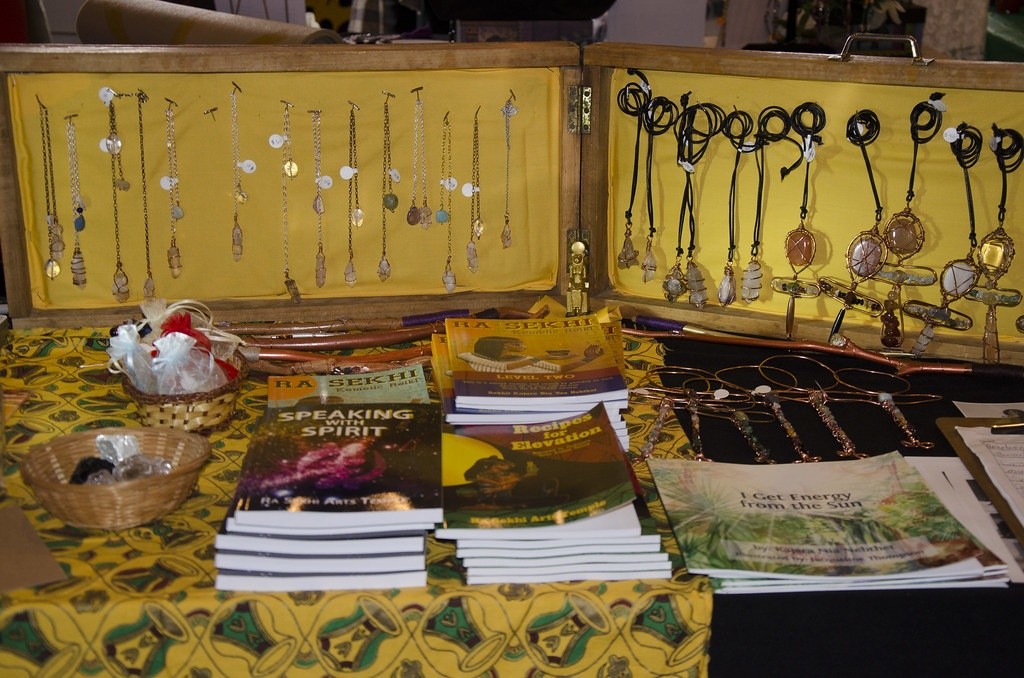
[18,425,213,532]
[121,352,249,433]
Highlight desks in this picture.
[0,306,1024,678]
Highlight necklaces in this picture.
[611,66,1024,365]
[466,116,484,273]
[502,101,518,250]
[165,108,183,281]
[376,103,398,283]
[406,101,432,229]
[436,118,456,292]
[67,116,85,290]
[229,89,246,262]
[99,91,155,304]
[282,106,303,306]
[346,110,364,288]
[310,113,326,289]
[37,103,64,283]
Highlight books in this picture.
[209,314,1017,596]
